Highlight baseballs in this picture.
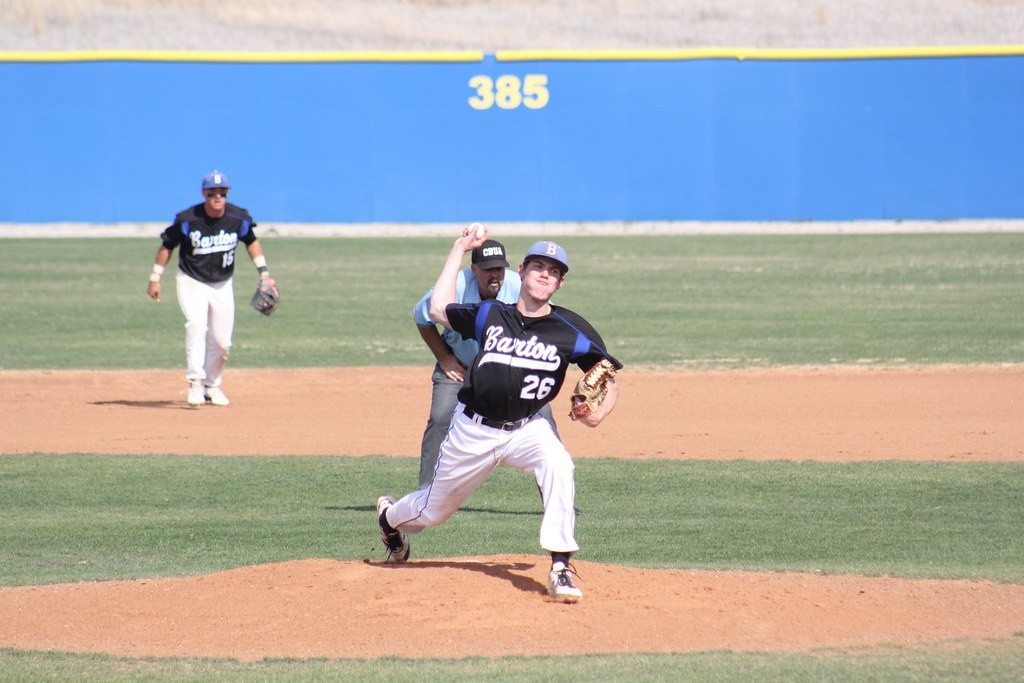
[468,222,485,239]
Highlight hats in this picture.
[202,170,230,189]
[523,240,569,273]
[472,240,510,269]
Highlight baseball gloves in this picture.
[567,357,620,428]
[249,278,280,316]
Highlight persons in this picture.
[376,222,623,603]
[146,170,280,406]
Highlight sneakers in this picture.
[376,494,411,564]
[547,561,586,603]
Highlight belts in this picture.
[463,406,534,430]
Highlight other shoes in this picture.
[205,387,230,406]
[186,380,206,405]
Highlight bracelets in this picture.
[149,264,164,282]
[253,255,269,276]
